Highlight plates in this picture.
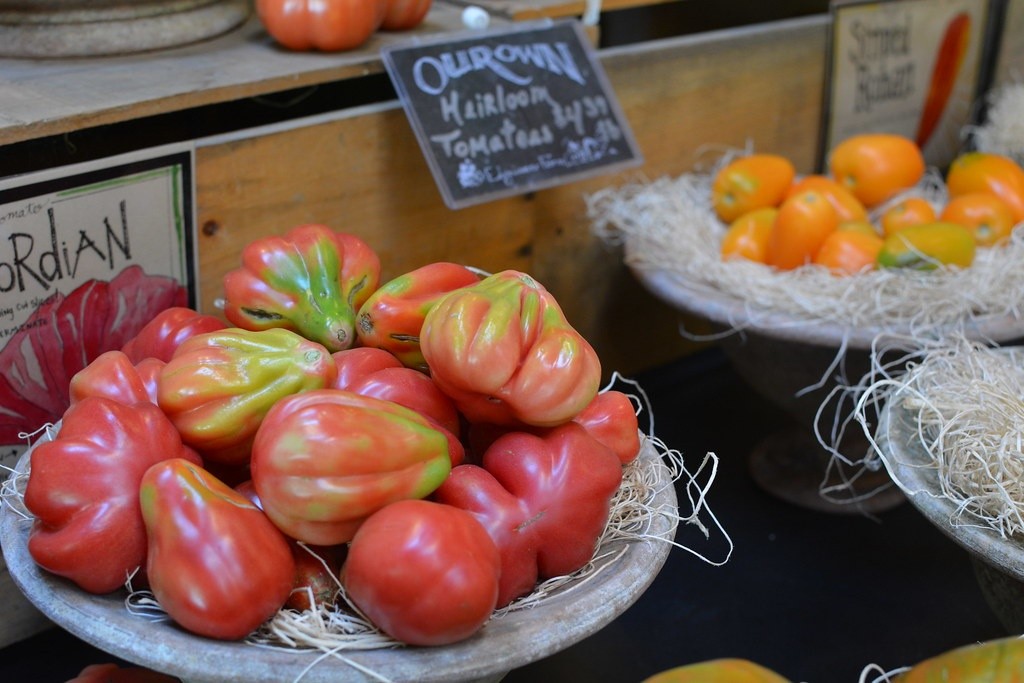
[0,419,678,683]
[621,236,1024,349]
[881,348,1024,576]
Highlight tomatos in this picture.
[710,135,1024,278]
[254,0,432,51]
[24,223,640,650]
